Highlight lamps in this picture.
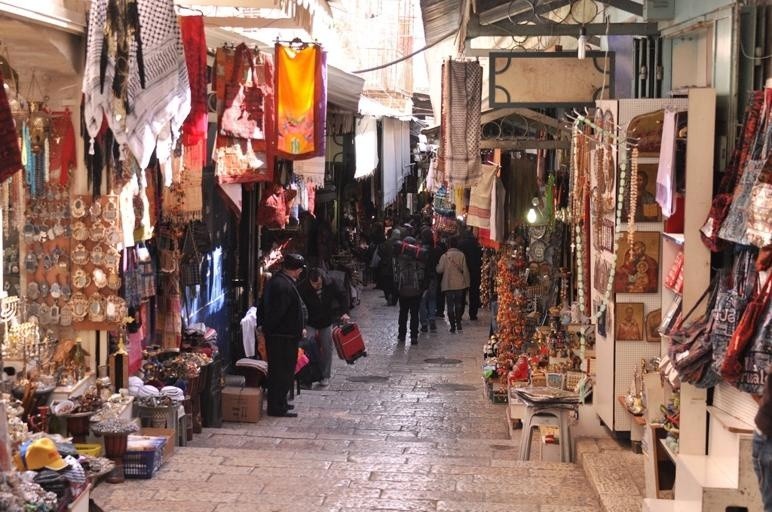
[526,158,537,224]
[578,0,586,60]
[532,184,540,206]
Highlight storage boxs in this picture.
[133,428,175,457]
[220,386,264,423]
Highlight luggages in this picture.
[330,319,368,364]
[298,338,325,386]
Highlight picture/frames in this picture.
[652,425,679,499]
[614,303,644,341]
[616,164,663,222]
[613,231,660,294]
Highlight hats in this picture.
[285,253,308,269]
[24,437,72,471]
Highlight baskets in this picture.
[123,438,167,480]
[74,442,103,457]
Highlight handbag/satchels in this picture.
[670,82,771,404]
[182,264,201,286]
[121,242,156,334]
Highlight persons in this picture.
[366,203,483,346]
[615,240,659,294]
[256,253,309,419]
[623,170,660,221]
[616,305,640,340]
[294,267,348,390]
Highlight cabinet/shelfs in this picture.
[661,88,714,499]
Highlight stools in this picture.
[133,397,180,446]
[518,406,573,462]
